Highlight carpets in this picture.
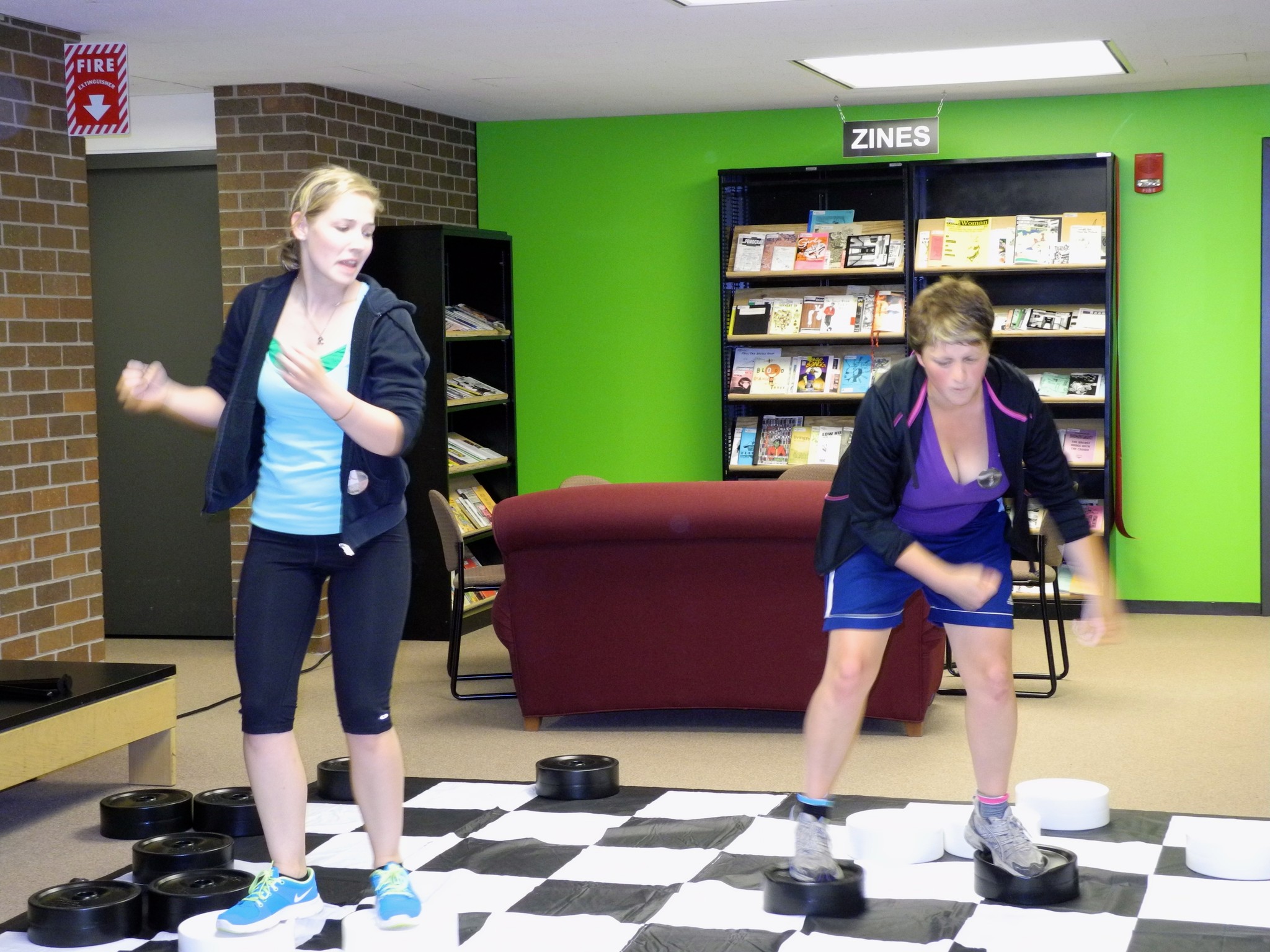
[0,773,1270,952]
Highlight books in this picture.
[448,437,505,466]
[729,210,1105,597]
[450,557,496,609]
[449,486,497,534]
[443,302,502,331]
[446,372,504,399]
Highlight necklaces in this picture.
[300,290,345,344]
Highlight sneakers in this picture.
[788,807,843,883]
[370,862,421,930]
[962,795,1045,879]
[217,860,323,934]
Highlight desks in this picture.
[0,660,179,790]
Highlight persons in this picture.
[115,164,430,934]
[790,279,1118,883]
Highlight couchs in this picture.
[492,482,946,736]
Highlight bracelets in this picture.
[332,397,356,421]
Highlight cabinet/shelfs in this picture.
[370,224,519,641]
[718,161,911,481]
[909,152,1119,620]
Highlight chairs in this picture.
[428,490,516,700]
[936,482,1069,698]
